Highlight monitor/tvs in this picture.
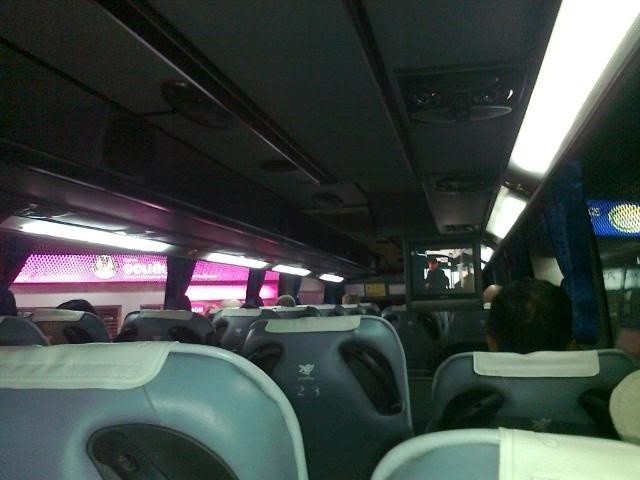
[404,233,484,314]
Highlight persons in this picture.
[486,277,573,354]
[424,257,449,291]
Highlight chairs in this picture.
[372,428,640,480]
[19,303,490,353]
[0,341,308,480]
[241,316,412,480]
[430,349,640,440]
